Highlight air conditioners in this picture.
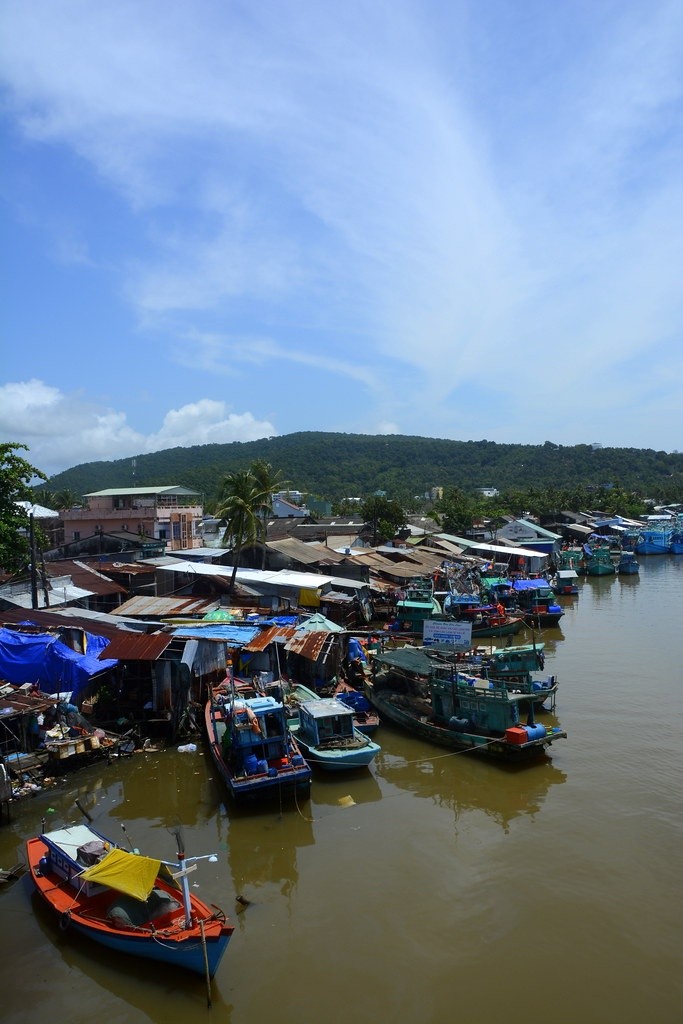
[95,525,102,530]
[145,530,151,535]
[121,525,128,530]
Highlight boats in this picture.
[199,660,318,812]
[268,639,383,778]
[24,815,231,985]
[1,515,682,771]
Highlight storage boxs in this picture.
[505,727,527,744]
[532,605,547,613]
[563,586,572,591]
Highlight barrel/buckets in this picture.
[549,606,561,612]
[39,855,51,874]
[522,723,546,741]
[244,752,258,775]
[257,760,268,773]
